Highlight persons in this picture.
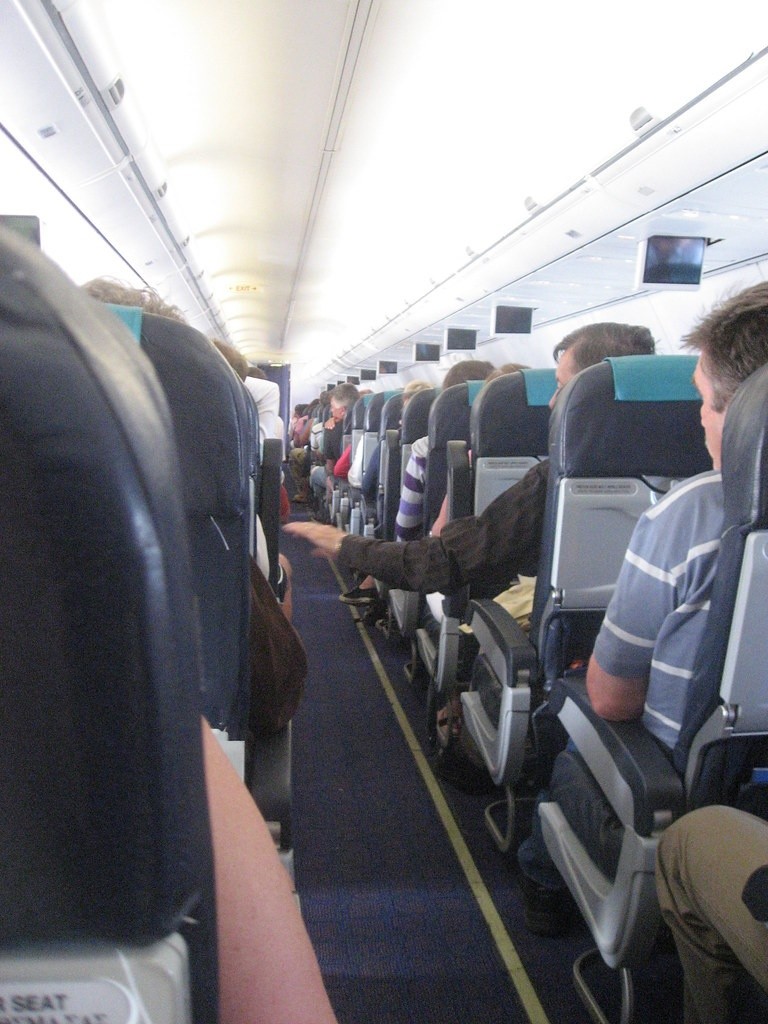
[82,276,768,1024]
[280,324,655,796]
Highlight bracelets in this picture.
[333,541,342,556]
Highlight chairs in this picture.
[0,225,766,1024]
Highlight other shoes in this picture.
[433,755,494,796]
[293,495,313,504]
[501,844,565,936]
[311,516,327,524]
[363,602,385,621]
[338,584,378,606]
